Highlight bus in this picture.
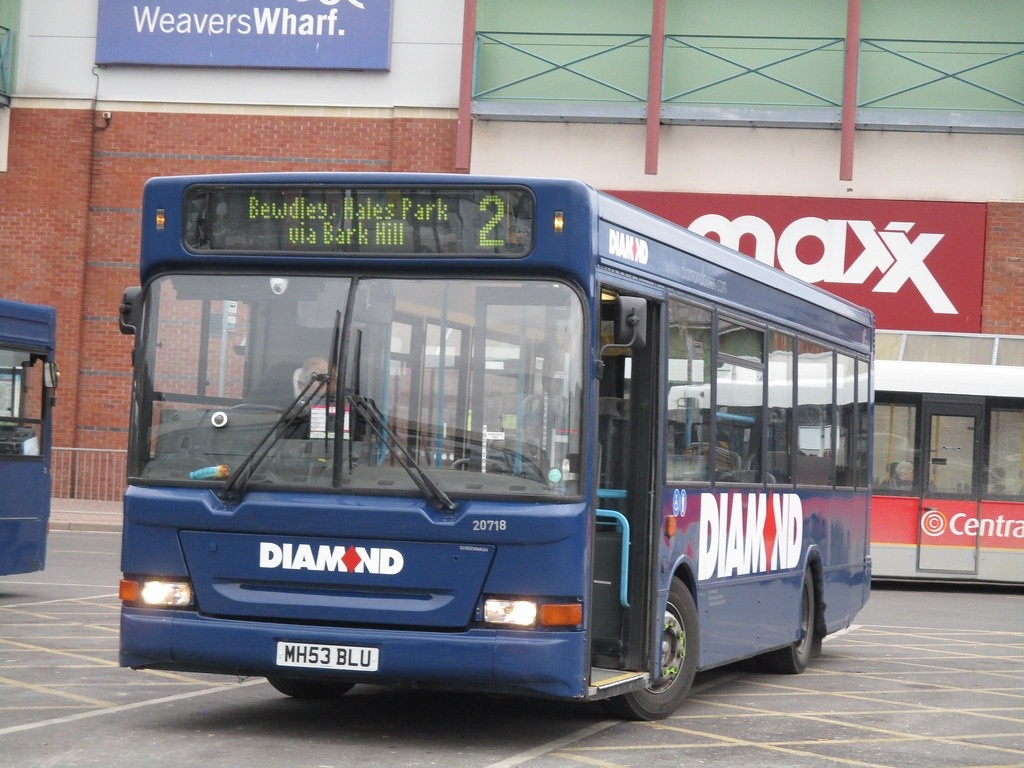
[0,300,59,573]
[559,331,1024,587]
[113,172,874,720]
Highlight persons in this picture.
[237,357,336,439]
[880,462,913,490]
[989,467,1010,494]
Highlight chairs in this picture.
[873,462,970,495]
[665,443,798,484]
